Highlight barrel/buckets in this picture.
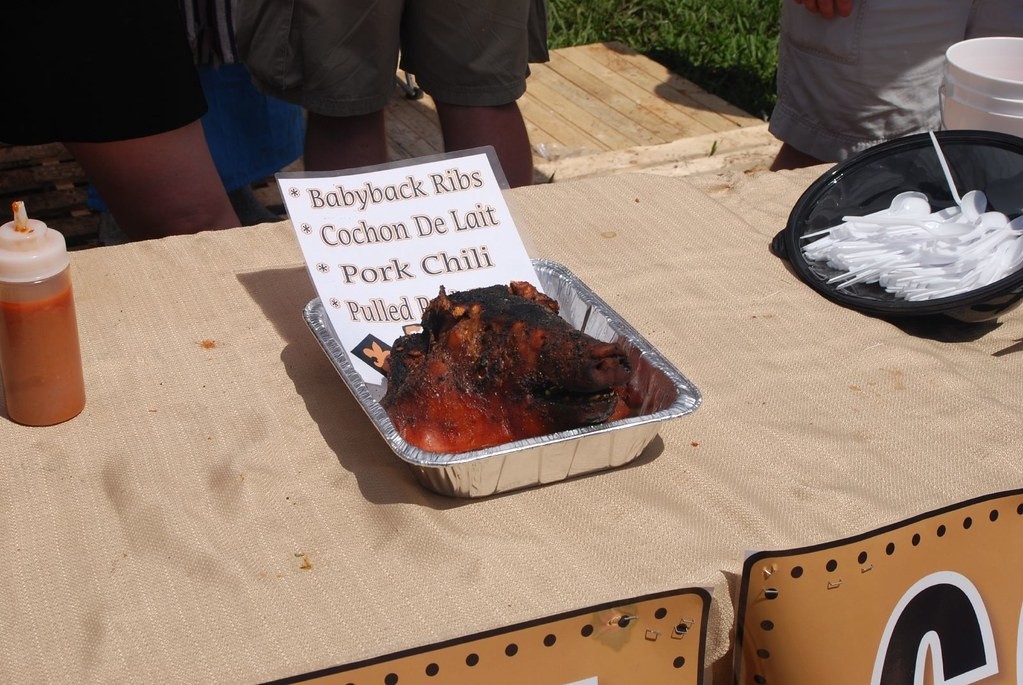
[938,37,1023,139]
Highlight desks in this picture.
[0,164,1023,685]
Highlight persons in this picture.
[237,0,550,189]
[87,0,306,225]
[768,0,1023,163]
[0,0,242,242]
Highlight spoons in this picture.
[799,130,1023,301]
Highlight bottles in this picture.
[0,201,85,426]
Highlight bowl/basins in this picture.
[301,259,702,496]
